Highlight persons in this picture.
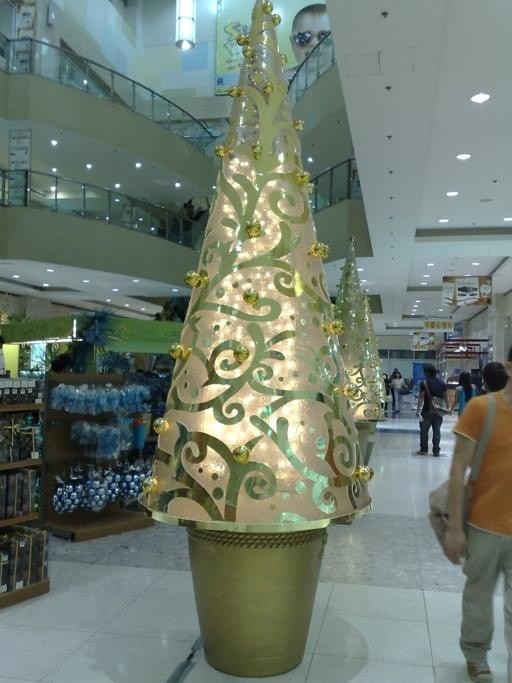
[443,344,512,682]
[156,197,196,242]
[416,364,453,456]
[457,369,487,396]
[48,349,167,422]
[482,360,507,393]
[281,3,332,102]
[380,366,409,415]
[448,372,479,418]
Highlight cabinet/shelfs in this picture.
[0,374,155,609]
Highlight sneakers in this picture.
[416,451,439,456]
[467,663,493,682]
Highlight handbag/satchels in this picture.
[421,396,449,420]
[428,479,468,558]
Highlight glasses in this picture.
[291,30,333,47]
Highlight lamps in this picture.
[174,0,197,52]
